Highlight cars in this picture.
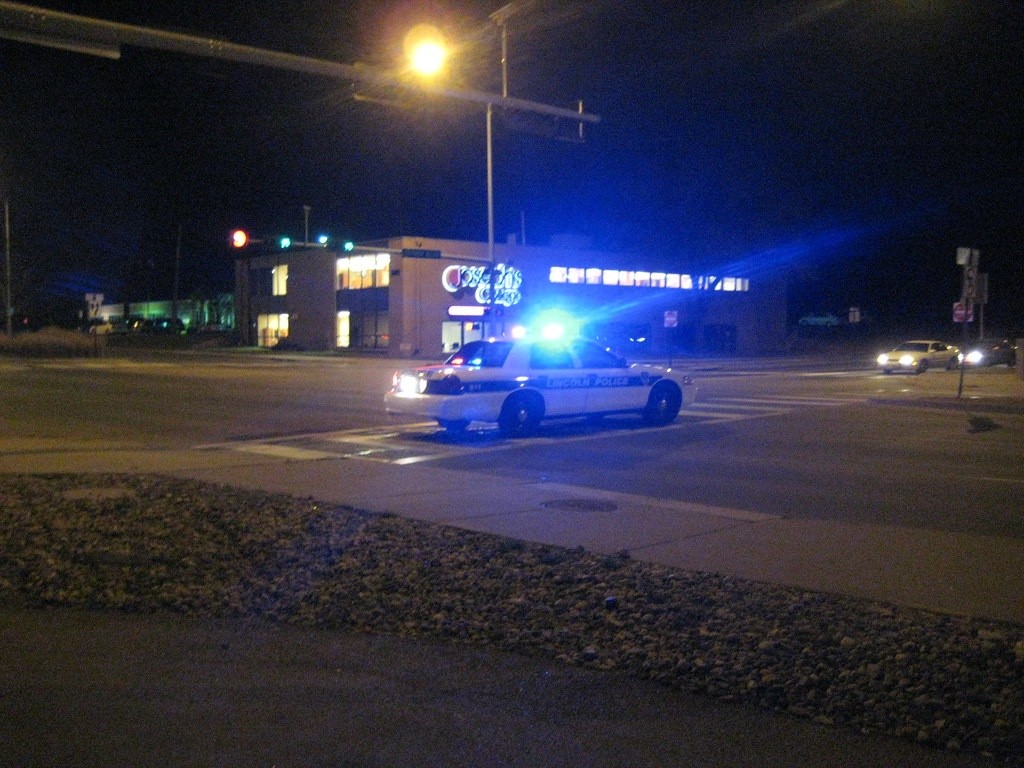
[74,315,228,336]
[877,339,961,375]
[382,312,698,437]
[947,340,1018,372]
